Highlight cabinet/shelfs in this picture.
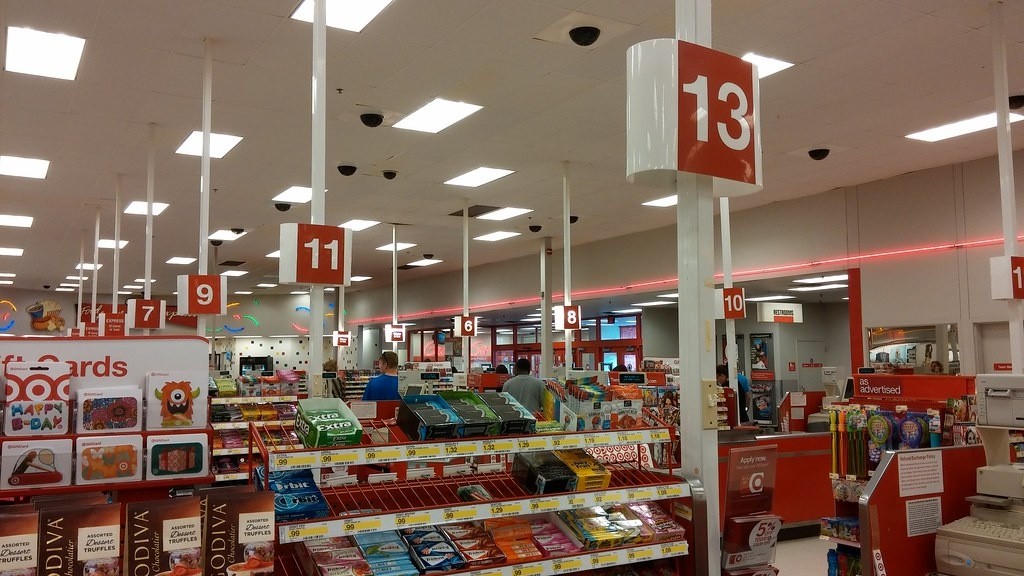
[117,363,979,576]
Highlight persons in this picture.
[738,373,750,405]
[502,359,545,412]
[931,361,944,373]
[716,365,750,423]
[965,428,977,446]
[362,352,400,401]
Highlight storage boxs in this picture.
[437,389,501,436]
[560,400,612,431]
[397,393,464,441]
[611,398,643,430]
[551,446,613,490]
[511,448,578,496]
[294,398,362,448]
[478,390,536,435]
